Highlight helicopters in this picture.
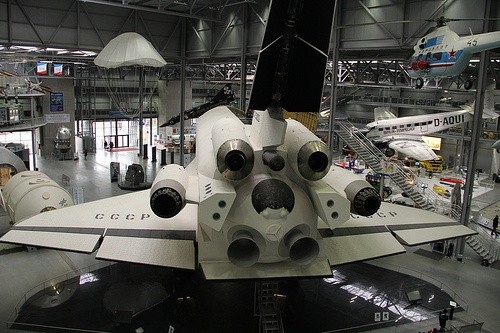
[335,1,500,91]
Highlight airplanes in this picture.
[365,98,500,162]
[0,0,480,280]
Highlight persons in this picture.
[109,142,113,151]
[104,141,107,146]
[489,214,499,236]
[83,150,87,159]
[346,154,375,171]
